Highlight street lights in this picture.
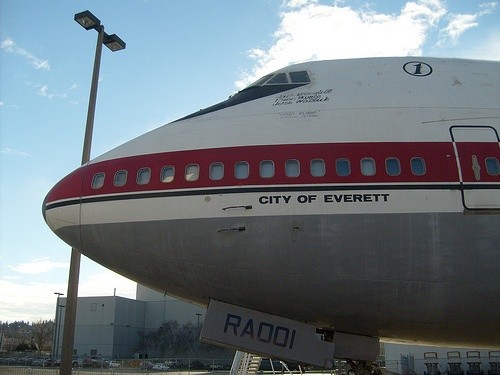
[51,293,65,367]
[56,10,128,375]
[195,313,202,325]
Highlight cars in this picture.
[1,356,224,372]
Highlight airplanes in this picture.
[42,54,500,365]
